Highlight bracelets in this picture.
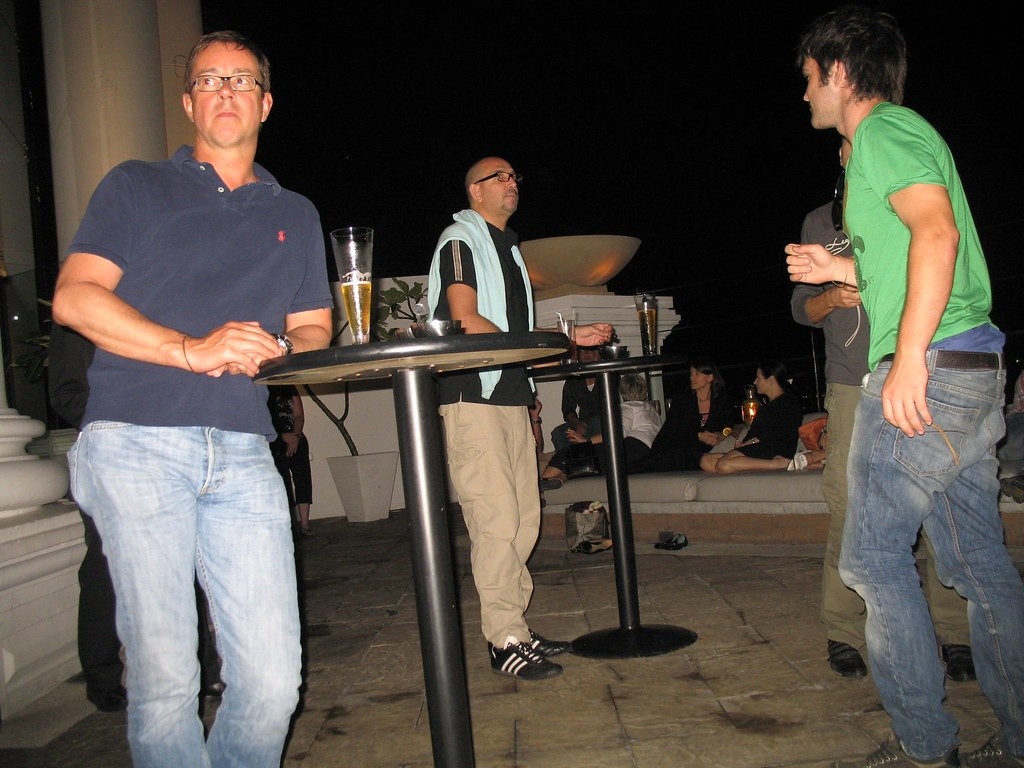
[532,417,542,424]
[296,434,301,438]
[832,255,848,288]
[182,336,197,373]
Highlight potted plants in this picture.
[302,276,428,522]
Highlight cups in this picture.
[638,309,656,356]
[557,320,576,364]
[330,226,374,344]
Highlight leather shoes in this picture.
[827,641,868,677]
[942,644,976,681]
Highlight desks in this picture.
[528,357,698,659]
[252,331,570,767]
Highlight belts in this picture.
[881,350,1005,370]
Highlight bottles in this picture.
[741,385,760,425]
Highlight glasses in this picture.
[189,75,266,93]
[832,170,845,231]
[474,172,524,184]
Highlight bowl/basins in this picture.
[396,320,467,339]
[598,345,627,359]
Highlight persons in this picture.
[796,5,1024,767]
[429,158,616,681]
[653,361,828,471]
[51,31,336,768]
[994,369,1024,504]
[789,133,979,682]
[78,370,321,712]
[527,350,664,509]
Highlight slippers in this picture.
[300,529,316,537]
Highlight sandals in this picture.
[579,539,612,554]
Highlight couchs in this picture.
[536,412,828,547]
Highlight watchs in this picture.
[270,333,295,356]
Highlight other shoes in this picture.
[1000,476,1024,503]
[86,685,129,711]
[787,459,795,472]
[538,477,563,492]
[198,683,226,699]
[794,450,811,471]
[654,534,688,551]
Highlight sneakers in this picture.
[523,628,571,656]
[957,739,1024,768]
[487,636,564,679]
[831,738,957,768]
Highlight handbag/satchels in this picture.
[564,501,610,552]
[797,416,827,451]
